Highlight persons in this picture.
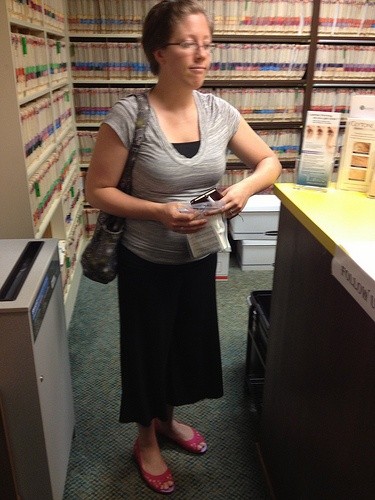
[85,0,282,494]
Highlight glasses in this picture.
[161,40,217,52]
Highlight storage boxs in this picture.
[229,194,281,241]
[215,252,231,282]
[235,240,277,272]
[244,289,270,432]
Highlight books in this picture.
[191,188,224,204]
[7,0,159,293]
[200,0,375,197]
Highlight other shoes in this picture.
[155,423,207,453]
[134,440,174,493]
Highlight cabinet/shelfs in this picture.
[67,0,375,238]
[0,1,85,330]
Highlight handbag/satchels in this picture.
[80,92,149,285]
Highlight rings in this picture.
[230,209,233,211]
[181,228,184,231]
